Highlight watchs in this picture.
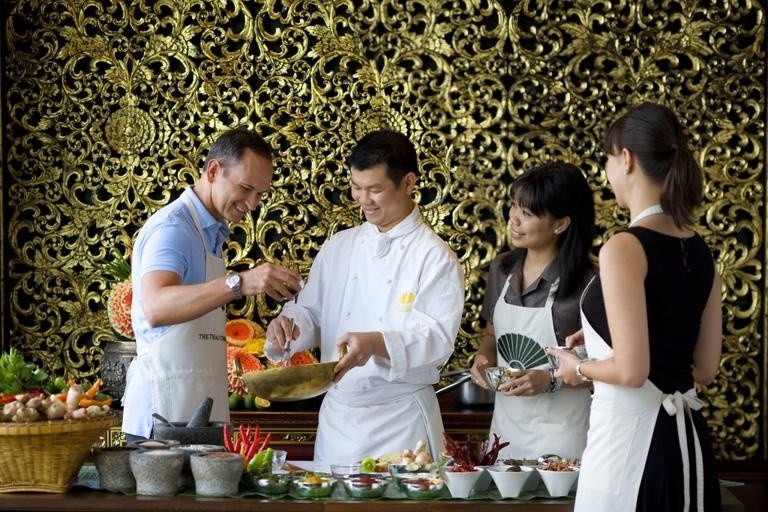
[224,268,248,304]
[544,368,567,394]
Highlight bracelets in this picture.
[575,358,591,382]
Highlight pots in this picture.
[232,341,351,405]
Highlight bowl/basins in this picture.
[254,473,296,494]
[289,478,338,498]
[342,479,388,498]
[545,344,591,368]
[387,463,431,477]
[329,463,366,479]
[398,477,444,502]
[264,450,287,472]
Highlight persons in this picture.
[477,162,602,479]
[543,99,729,512]
[111,130,302,467]
[262,126,465,473]
